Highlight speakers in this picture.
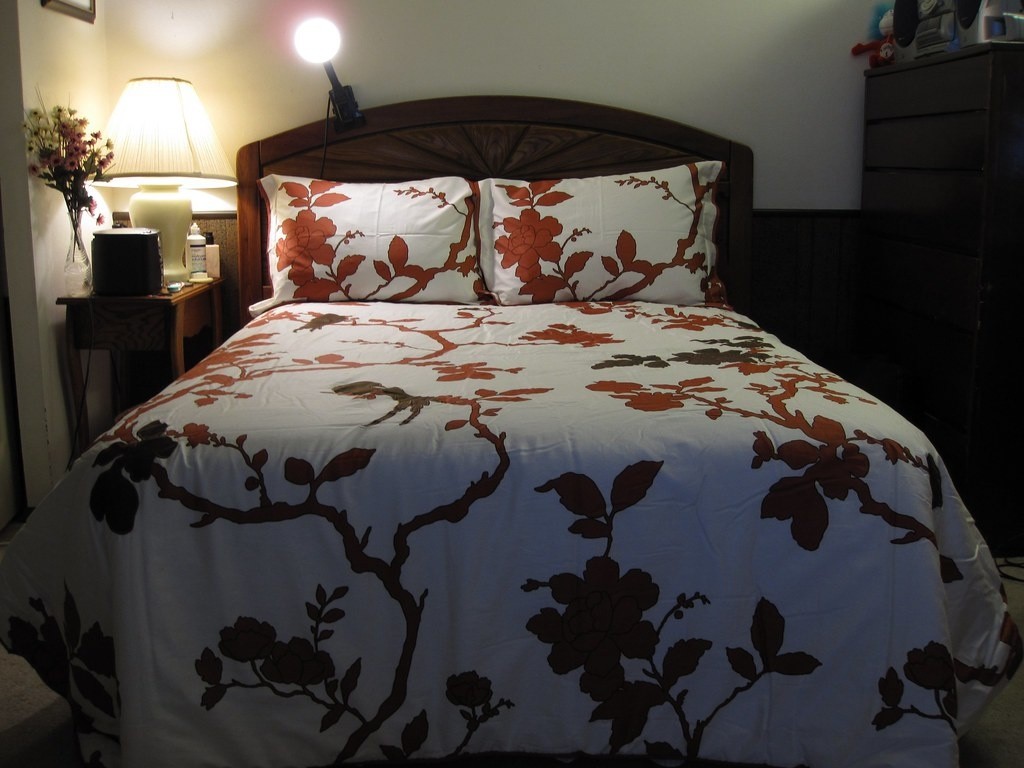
[894,0,920,62]
[956,0,1022,48]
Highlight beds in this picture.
[1,94,1024,768]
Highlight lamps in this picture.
[85,76,239,283]
[293,17,367,134]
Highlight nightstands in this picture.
[56,276,225,457]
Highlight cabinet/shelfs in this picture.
[854,42,1024,560]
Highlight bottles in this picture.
[185,224,207,280]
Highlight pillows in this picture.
[472,160,734,311]
[248,173,488,318]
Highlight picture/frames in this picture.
[41,0,96,24]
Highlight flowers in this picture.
[21,105,114,262]
